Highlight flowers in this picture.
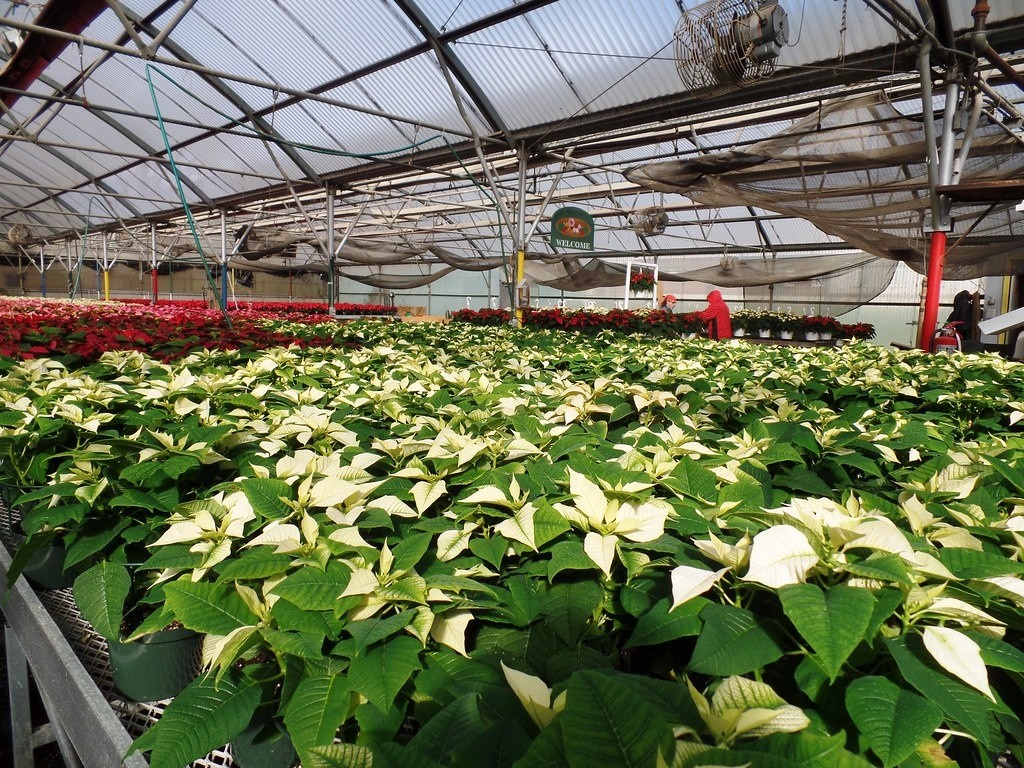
[629,271,658,297]
[0,296,1024,768]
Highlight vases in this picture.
[700,327,708,333]
[758,328,771,338]
[834,338,845,347]
[0,476,50,510]
[635,289,649,298]
[687,332,697,340]
[232,707,302,768]
[780,329,795,340]
[675,332,686,339]
[804,331,819,341]
[820,331,833,340]
[10,521,95,590]
[104,624,205,704]
[744,329,751,335]
[733,328,744,337]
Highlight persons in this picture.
[947,290,983,339]
[698,290,732,340]
[660,295,676,315]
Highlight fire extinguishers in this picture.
[929,321,965,353]
